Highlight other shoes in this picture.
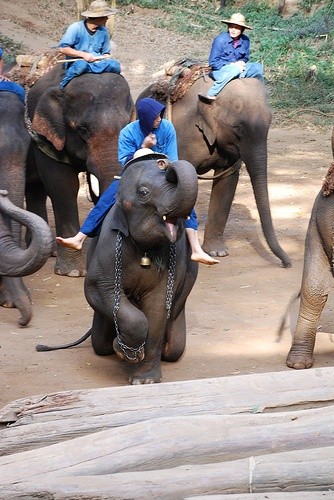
[198,93,215,100]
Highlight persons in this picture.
[0,47,26,105]
[56,97,220,265]
[57,0,121,90]
[207,13,266,100]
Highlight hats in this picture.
[125,147,168,168]
[221,12,253,30]
[81,0,119,17]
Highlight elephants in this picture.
[286,162,334,370]
[25,61,140,278]
[136,62,293,269]
[36,157,199,386]
[1,80,54,327]
